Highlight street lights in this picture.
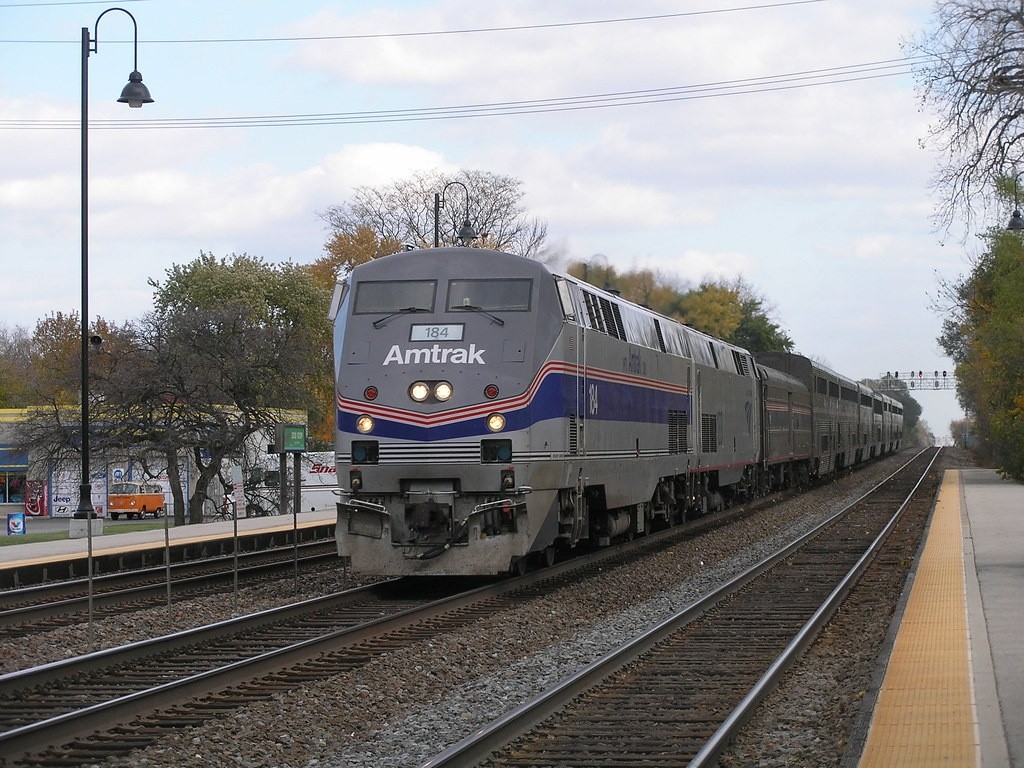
[432,180,480,249]
[78,6,155,521]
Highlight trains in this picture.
[327,245,909,586]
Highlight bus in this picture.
[108,481,165,520]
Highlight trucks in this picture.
[245,451,339,517]
